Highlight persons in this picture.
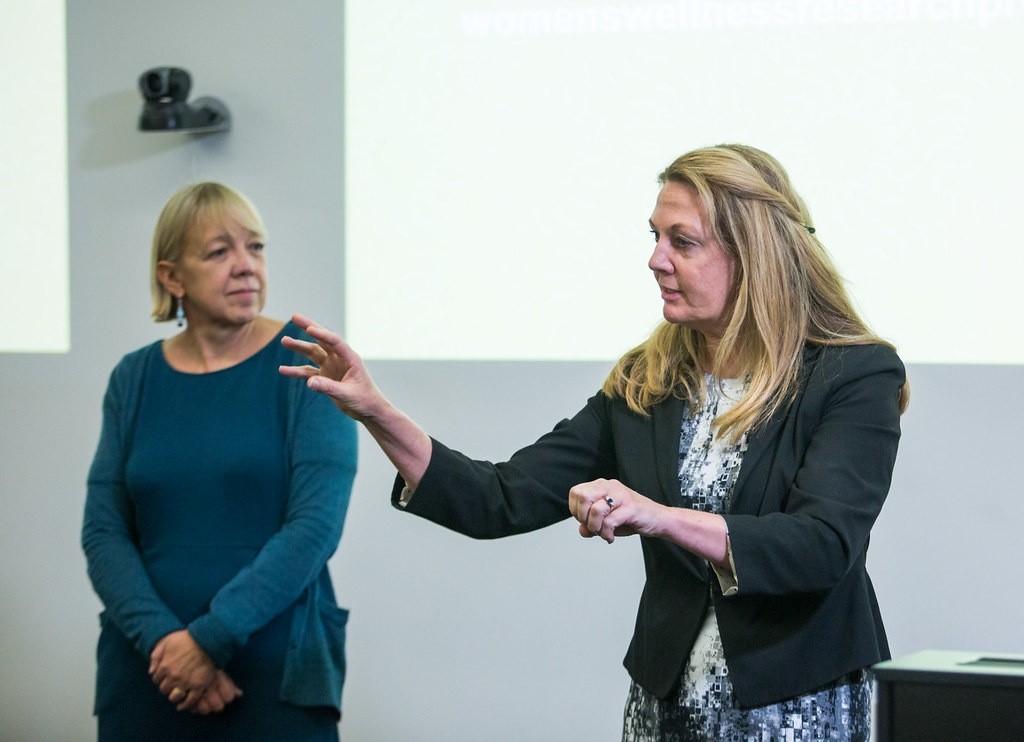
[280,145,910,742]
[81,182,358,742]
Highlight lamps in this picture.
[136,68,228,136]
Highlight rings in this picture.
[603,496,617,512]
